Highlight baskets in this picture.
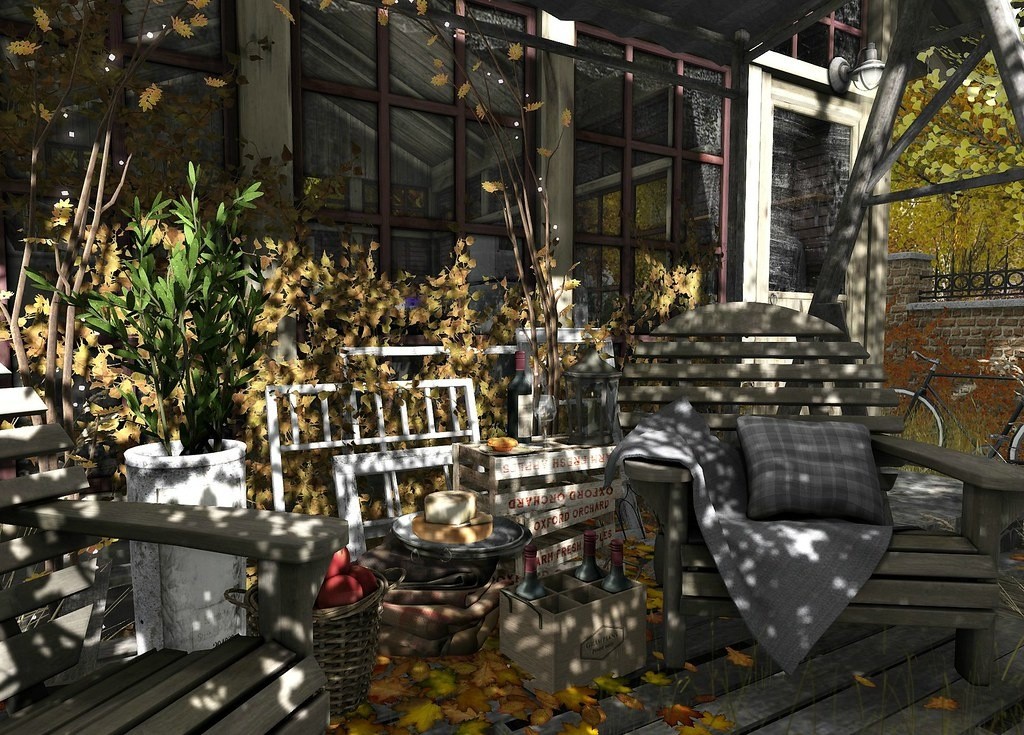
[224,568,412,717]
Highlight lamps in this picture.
[247,32,274,63]
[828,42,887,96]
[562,343,623,445]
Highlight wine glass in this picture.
[533,394,557,448]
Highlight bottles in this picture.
[575,529,633,594]
[508,350,533,444]
[515,546,545,600]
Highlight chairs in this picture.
[617,301,1024,686]
[0,365,352,735]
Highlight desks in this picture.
[390,511,536,587]
[448,435,622,577]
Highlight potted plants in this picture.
[22,160,270,657]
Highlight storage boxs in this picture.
[499,565,649,698]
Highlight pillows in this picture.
[735,413,889,527]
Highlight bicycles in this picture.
[885,348,1024,468]
